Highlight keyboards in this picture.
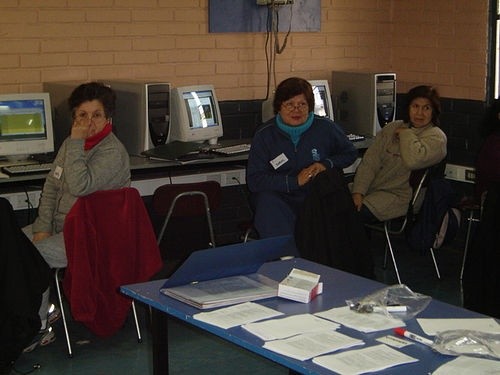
[211,144,252,155]
[2,163,53,176]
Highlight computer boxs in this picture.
[99,78,172,157]
[331,68,397,137]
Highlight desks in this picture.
[119,257,500,375]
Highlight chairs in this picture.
[148,180,223,334]
[358,164,446,285]
[235,166,356,270]
[50,187,150,360]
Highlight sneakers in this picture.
[22,320,57,354]
[46,301,62,326]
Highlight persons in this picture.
[23,82,130,351]
[246,78,357,238]
[347,85,449,224]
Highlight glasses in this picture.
[282,102,309,112]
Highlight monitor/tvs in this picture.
[262,80,335,124]
[0,92,55,167]
[170,85,223,146]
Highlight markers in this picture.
[366,306,407,311]
[395,327,434,346]
